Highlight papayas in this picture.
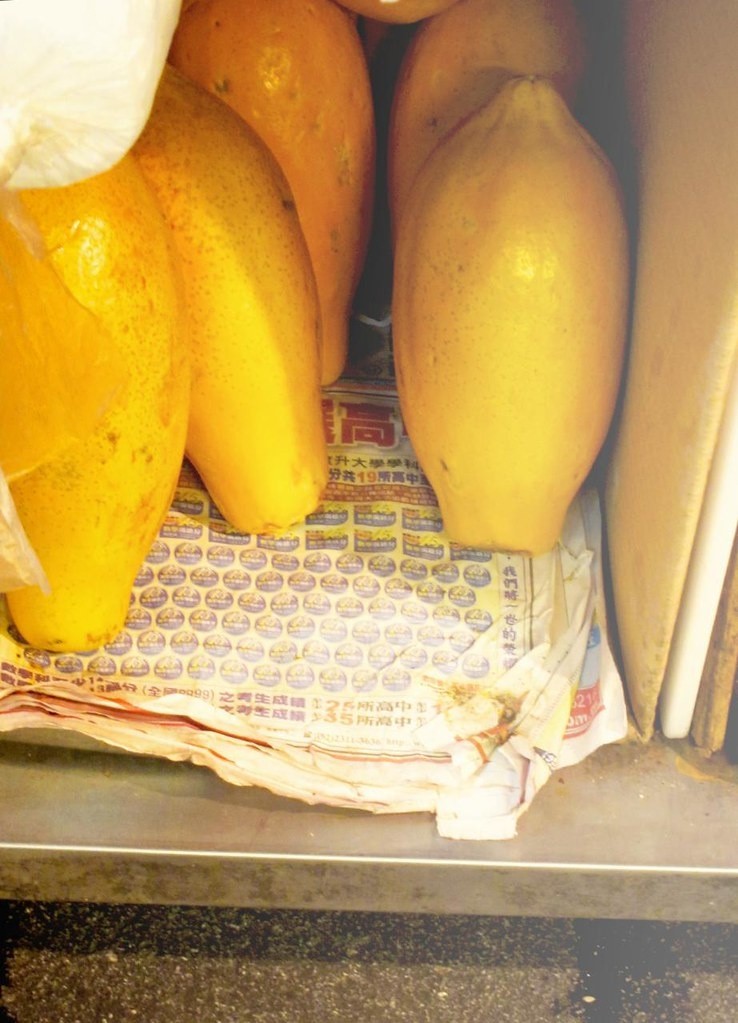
[0,0,632,648]
